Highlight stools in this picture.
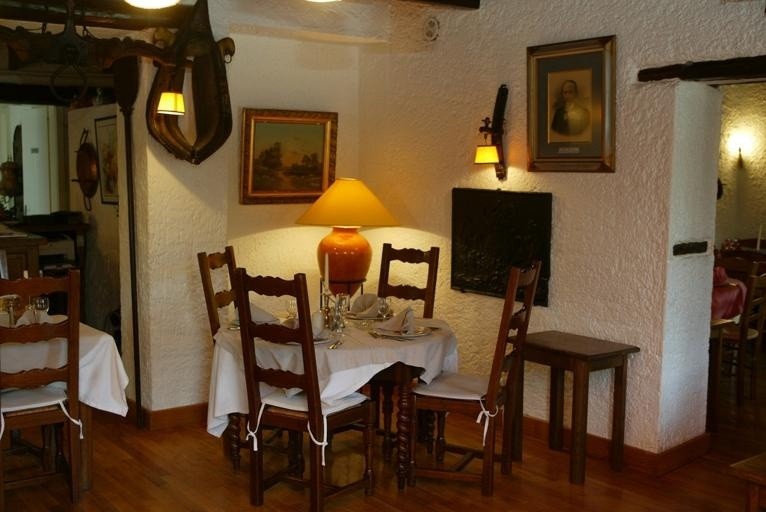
[505,331,640,484]
[710,318,735,433]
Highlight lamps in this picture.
[294,177,401,298]
[475,83,510,179]
[156,57,188,116]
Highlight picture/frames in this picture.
[526,35,618,173]
[95,115,119,205]
[238,106,339,206]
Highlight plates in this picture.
[374,325,431,337]
[346,316,389,319]
[47,315,67,324]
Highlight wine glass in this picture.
[3,296,19,327]
[285,298,296,319]
[333,291,351,338]
[29,296,49,323]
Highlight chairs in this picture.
[0,270,81,504]
[235,267,374,512]
[0,226,48,282]
[406,261,541,495]
[198,245,295,467]
[326,243,439,463]
[711,273,766,412]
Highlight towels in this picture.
[231,300,279,325]
[373,307,415,335]
[348,293,377,318]
[13,309,51,327]
[281,318,299,345]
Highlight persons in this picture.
[549,80,590,136]
